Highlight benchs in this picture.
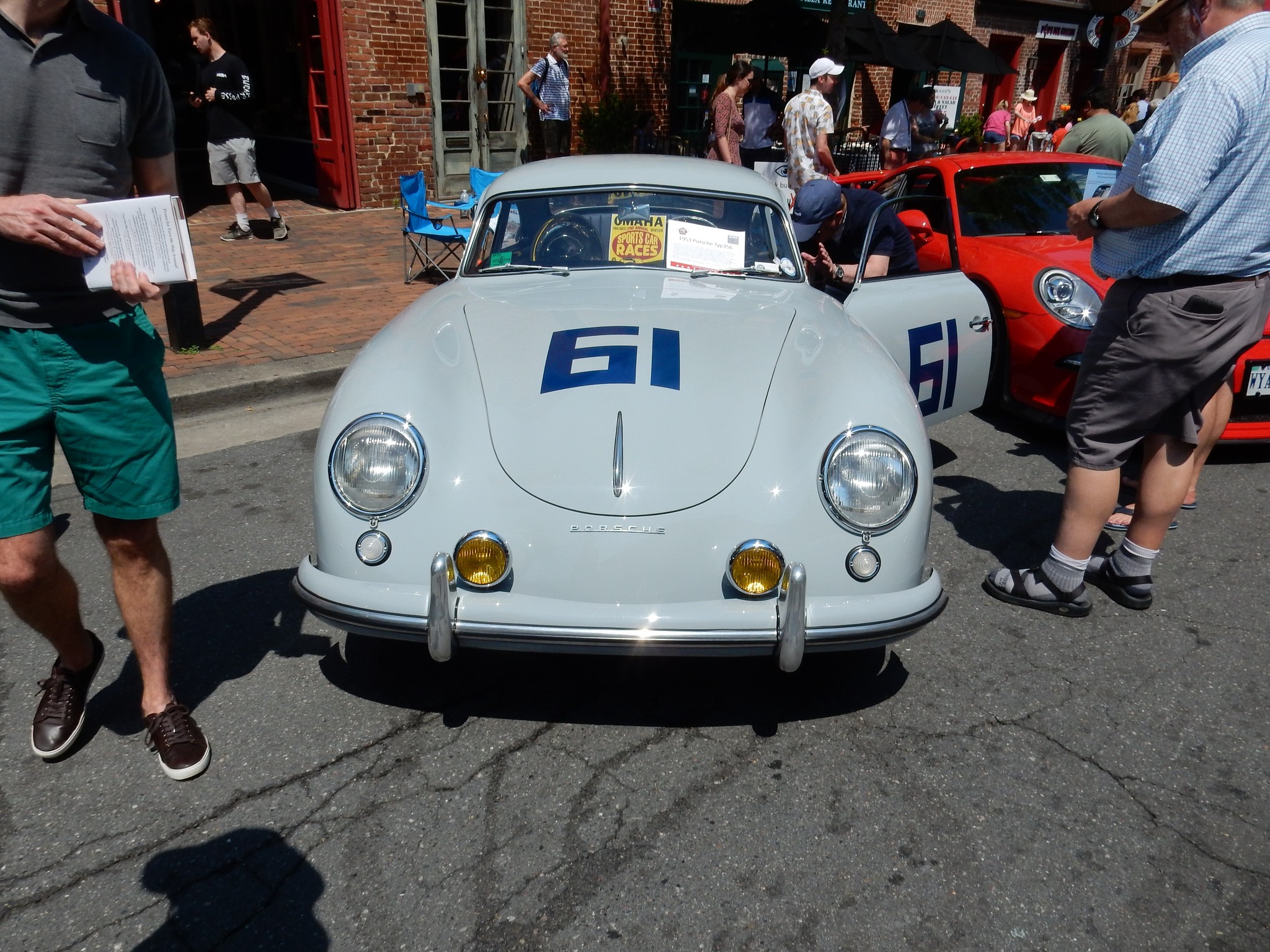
[553,205,730,262]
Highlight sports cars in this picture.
[828,154,1270,454]
[290,152,998,672]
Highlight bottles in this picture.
[460,189,470,219]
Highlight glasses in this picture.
[1159,0,1186,34]
[744,77,756,86]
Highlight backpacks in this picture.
[526,58,567,115]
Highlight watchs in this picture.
[831,263,844,284]
[1088,200,1111,230]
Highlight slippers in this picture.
[1121,480,1197,509]
[1103,508,1178,530]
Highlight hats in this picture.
[809,58,845,80]
[1132,0,1168,26]
[1020,89,1037,102]
[791,179,841,242]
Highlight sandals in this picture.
[985,565,1093,618]
[1083,548,1153,609]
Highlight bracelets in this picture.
[944,120,948,124]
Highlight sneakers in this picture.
[270,212,288,239]
[30,629,105,757]
[142,693,211,782]
[220,225,255,241]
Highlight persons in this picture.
[1055,84,1135,163]
[878,89,927,214]
[1102,369,1234,529]
[741,66,786,170]
[0,0,213,782]
[907,85,949,161]
[1009,90,1038,152]
[517,33,572,159]
[1041,101,1082,153]
[1112,88,1166,135]
[701,60,754,168]
[187,17,287,241]
[982,0,1270,618]
[982,100,1011,152]
[789,180,919,304]
[780,57,845,196]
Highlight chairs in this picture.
[466,165,519,260]
[397,171,492,286]
